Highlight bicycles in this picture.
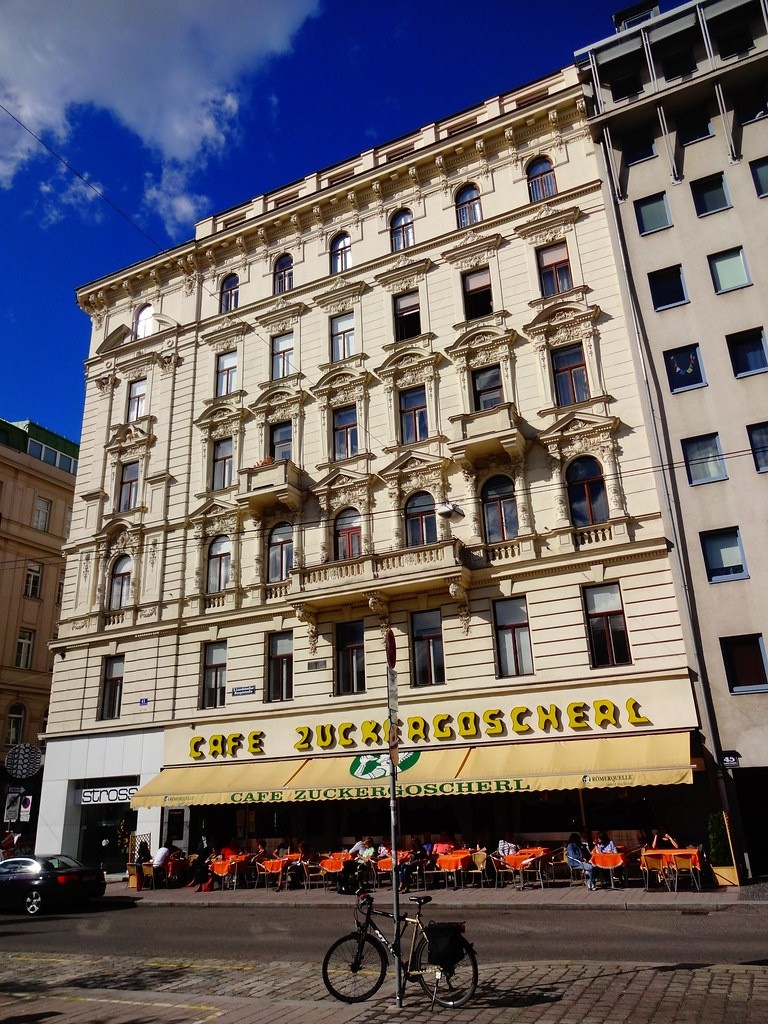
[321,863,479,1011]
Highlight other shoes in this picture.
[587,882,596,891]
[398,884,409,894]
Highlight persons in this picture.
[137,836,183,888]
[454,833,521,870]
[203,836,317,891]
[342,829,453,895]
[567,832,618,890]
[647,826,679,850]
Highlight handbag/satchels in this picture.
[427,922,465,967]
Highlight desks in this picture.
[259,859,291,891]
[502,854,535,891]
[376,856,407,888]
[282,853,304,862]
[425,854,472,892]
[639,848,703,890]
[228,853,255,862]
[448,849,478,854]
[318,860,346,890]
[516,848,554,880]
[209,860,247,892]
[330,852,358,861]
[588,852,629,891]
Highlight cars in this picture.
[0,854,106,916]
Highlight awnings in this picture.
[131,732,696,811]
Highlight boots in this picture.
[186,880,195,887]
[194,883,202,892]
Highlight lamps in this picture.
[437,502,466,524]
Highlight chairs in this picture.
[127,843,704,892]
